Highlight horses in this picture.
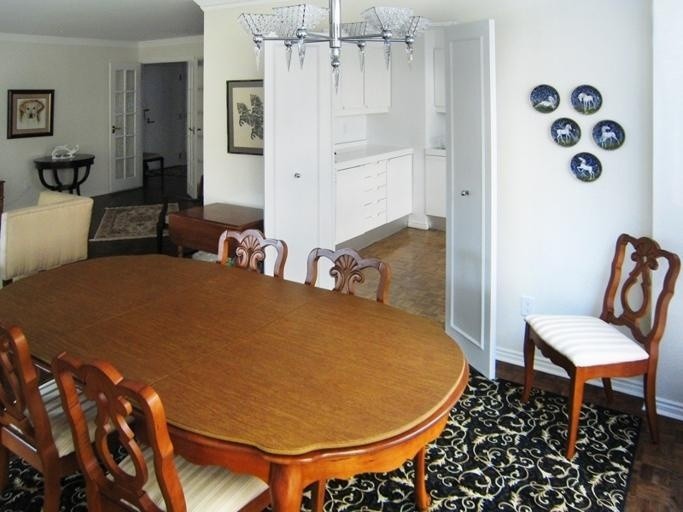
[576,91,595,111]
[535,93,556,110]
[598,126,619,146]
[555,124,572,143]
[577,157,595,178]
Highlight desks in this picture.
[33,152,96,196]
[167,202,263,259]
[0,254,471,512]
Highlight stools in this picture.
[144,153,165,188]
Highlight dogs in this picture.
[18,99,43,129]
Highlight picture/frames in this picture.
[570,85,602,115]
[226,79,264,156]
[592,120,625,152]
[530,85,561,114]
[571,151,602,182]
[550,118,582,148]
[6,88,55,139]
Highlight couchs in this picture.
[0,189,93,286]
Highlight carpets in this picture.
[90,203,179,243]
[0,363,644,512]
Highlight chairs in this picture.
[51,350,327,512]
[215,230,288,280]
[522,233,682,460]
[303,247,390,307]
[0,321,137,512]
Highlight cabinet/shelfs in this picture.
[433,47,446,112]
[334,40,392,116]
[334,153,413,252]
[425,155,447,231]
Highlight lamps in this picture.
[238,0,429,97]
